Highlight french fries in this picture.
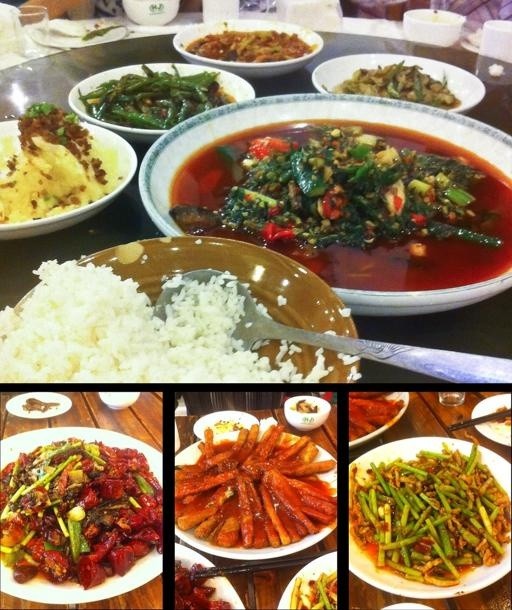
[174,424,337,548]
[349,391,404,443]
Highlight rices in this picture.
[1,255,363,384]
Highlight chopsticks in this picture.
[183,555,324,580]
[444,408,509,431]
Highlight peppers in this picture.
[77,64,221,130]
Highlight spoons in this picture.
[155,269,509,384]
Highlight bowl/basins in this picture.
[282,396,333,429]
[120,0,183,26]
[140,93,511,316]
[99,391,140,409]
[402,8,463,45]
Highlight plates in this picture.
[1,427,163,604]
[349,435,510,600]
[5,233,360,380]
[313,52,487,112]
[349,392,412,446]
[1,116,137,241]
[31,16,130,50]
[67,60,257,141]
[192,409,259,437]
[174,431,338,562]
[468,395,511,447]
[7,392,74,421]
[174,543,246,609]
[175,21,321,73]
[275,550,337,609]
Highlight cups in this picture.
[437,389,464,406]
[480,19,511,65]
[202,0,240,22]
[9,6,55,57]
